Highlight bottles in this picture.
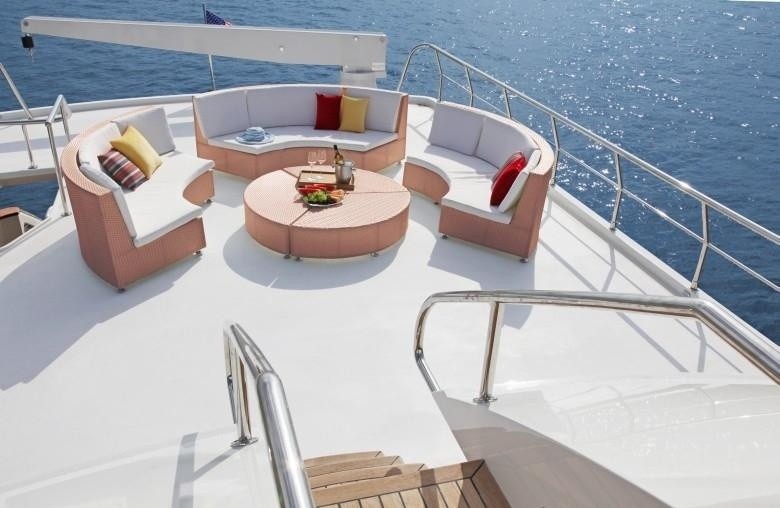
[332,145,345,166]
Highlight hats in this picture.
[235,127,275,146]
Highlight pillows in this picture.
[78,107,177,238]
[428,102,542,213]
[193,84,403,133]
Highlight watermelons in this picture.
[296,183,336,195]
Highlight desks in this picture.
[243,164,411,261]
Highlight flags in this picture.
[202,1,234,28]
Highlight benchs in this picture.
[60,104,216,294]
[403,100,554,262]
[191,82,409,178]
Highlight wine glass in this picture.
[307,151,317,180]
[317,148,326,180]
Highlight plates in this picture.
[303,191,347,208]
